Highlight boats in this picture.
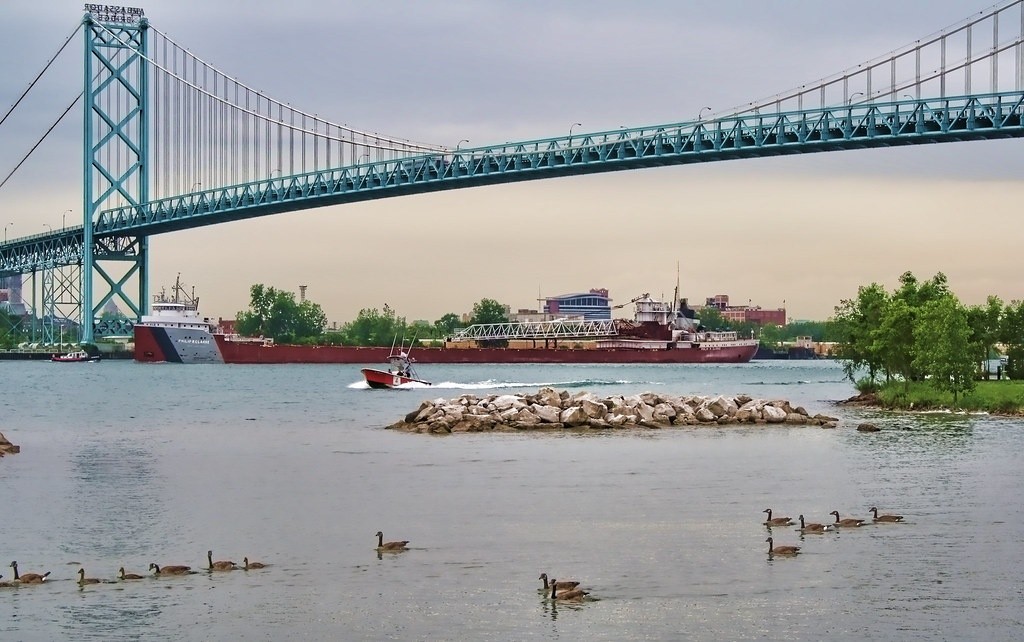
[359,330,432,389]
[133,270,760,364]
[51,349,102,362]
[984,353,1009,375]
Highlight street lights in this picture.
[270,169,282,179]
[43,223,52,238]
[358,154,369,165]
[63,210,73,229]
[457,140,469,151]
[5,222,14,244]
[904,95,915,111]
[191,182,201,193]
[60,324,64,352]
[699,107,712,120]
[849,92,864,116]
[620,125,629,129]
[570,123,582,135]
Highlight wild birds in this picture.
[9,560,52,584]
[869,506,904,523]
[797,514,834,533]
[830,510,865,528]
[538,572,591,602]
[243,557,266,570]
[766,536,802,554]
[119,567,143,580]
[76,568,100,584]
[208,550,237,570]
[375,530,408,550]
[764,508,792,526]
[148,563,191,575]
